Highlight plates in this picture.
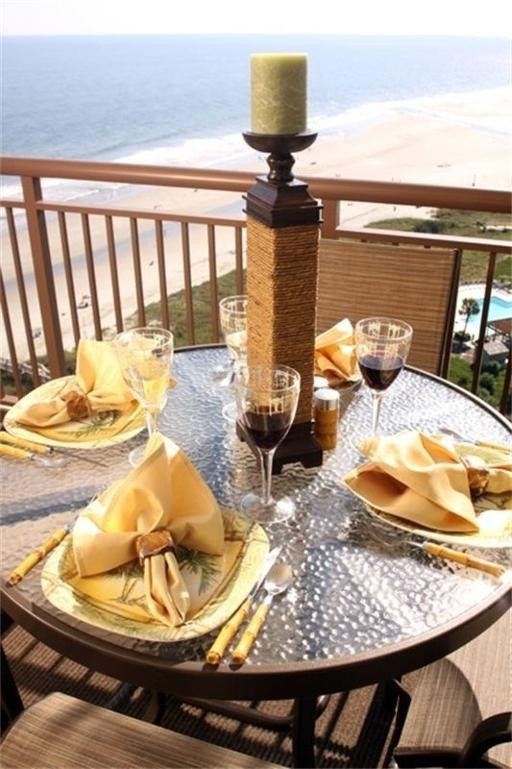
[364,436,512,552]
[3,374,165,451]
[328,378,362,394]
[42,506,272,645]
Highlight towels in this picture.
[338,425,511,534]
[312,315,360,382]
[12,334,180,434]
[70,435,225,630]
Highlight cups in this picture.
[218,295,249,369]
[114,326,178,469]
[352,317,413,453]
[233,365,300,523]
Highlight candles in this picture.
[251,53,306,134]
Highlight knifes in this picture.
[205,544,284,667]
[1,430,110,468]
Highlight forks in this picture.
[346,514,506,578]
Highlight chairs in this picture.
[394,604,512,769]
[314,239,462,377]
[1,693,294,769]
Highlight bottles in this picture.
[314,389,339,450]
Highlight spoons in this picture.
[0,445,68,469]
[232,563,293,666]
